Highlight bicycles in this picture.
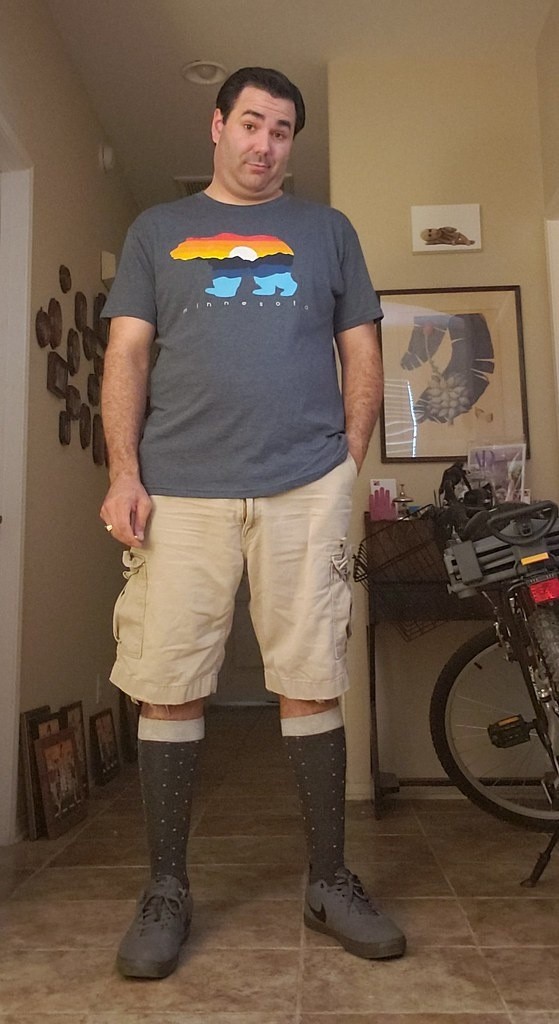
[427,454,559,887]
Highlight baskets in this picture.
[354,504,460,642]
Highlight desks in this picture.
[360,512,559,820]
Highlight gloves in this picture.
[369,487,397,522]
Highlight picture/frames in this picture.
[20,689,142,840]
[34,264,152,469]
[375,285,531,465]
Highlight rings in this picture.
[105,525,113,532]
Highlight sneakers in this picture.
[303,868,407,959]
[116,875,194,977]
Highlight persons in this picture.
[99,65,409,979]
